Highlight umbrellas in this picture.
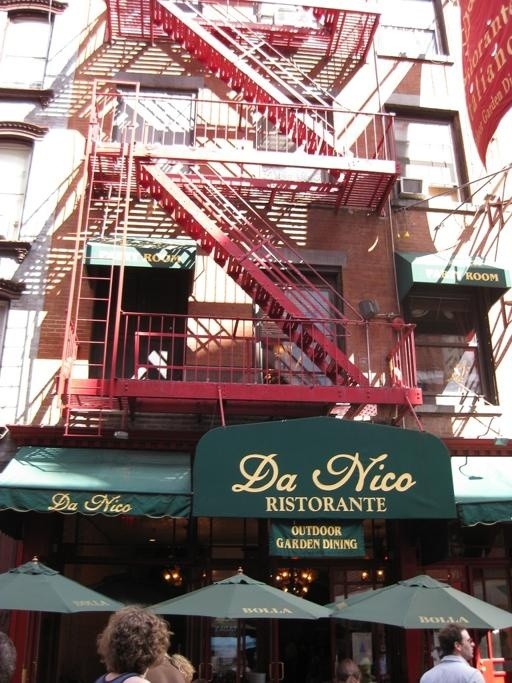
[1,553,127,683]
[145,565,336,683]
[321,572,512,682]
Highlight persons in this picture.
[336,657,362,683]
[92,599,175,682]
[418,622,484,683]
[0,631,19,682]
[146,654,186,683]
[170,652,197,682]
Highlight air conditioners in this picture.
[399,177,424,199]
